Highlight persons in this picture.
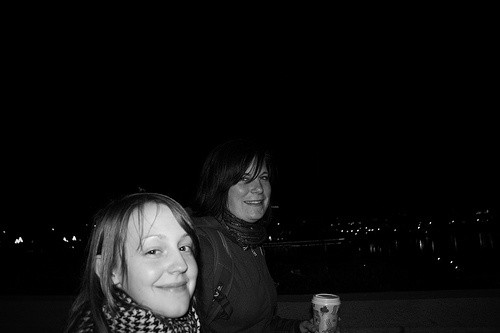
[184,140,344,332]
[62,190,205,333]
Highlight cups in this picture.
[311,293,341,333]
